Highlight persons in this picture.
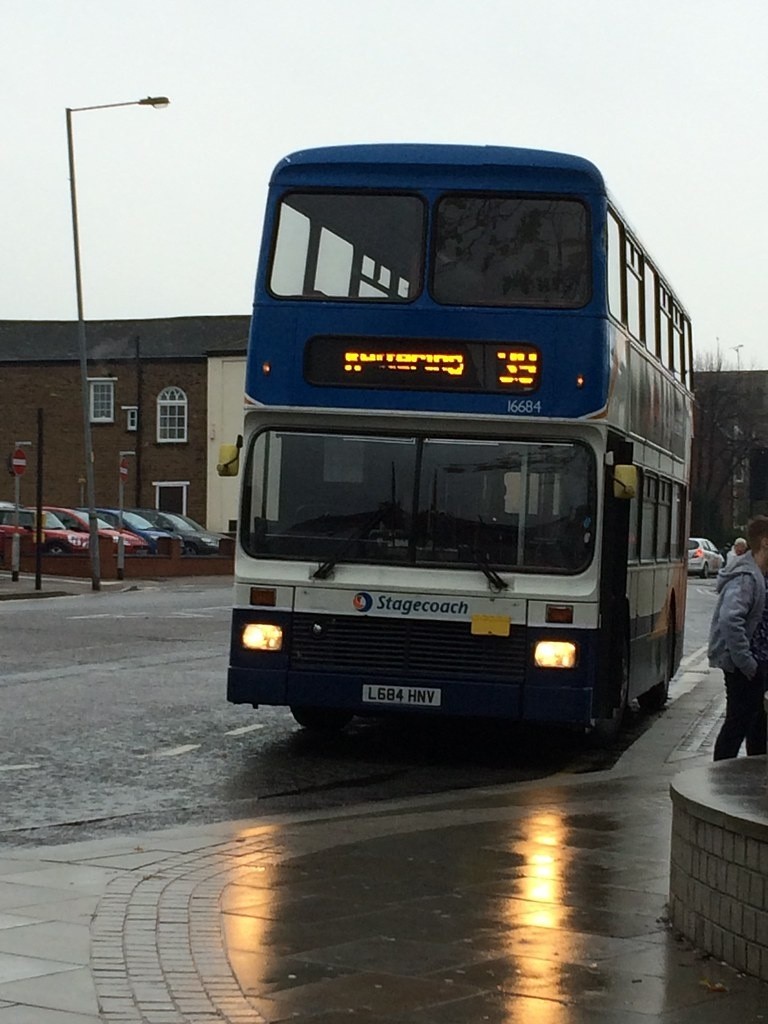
[725,537,748,566]
[708,515,768,762]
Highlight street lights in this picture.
[66,96,168,587]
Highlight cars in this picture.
[687,538,724,579]
[0,503,220,557]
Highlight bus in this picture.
[218,143,694,741]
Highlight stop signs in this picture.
[120,459,129,481]
[11,449,26,475]
